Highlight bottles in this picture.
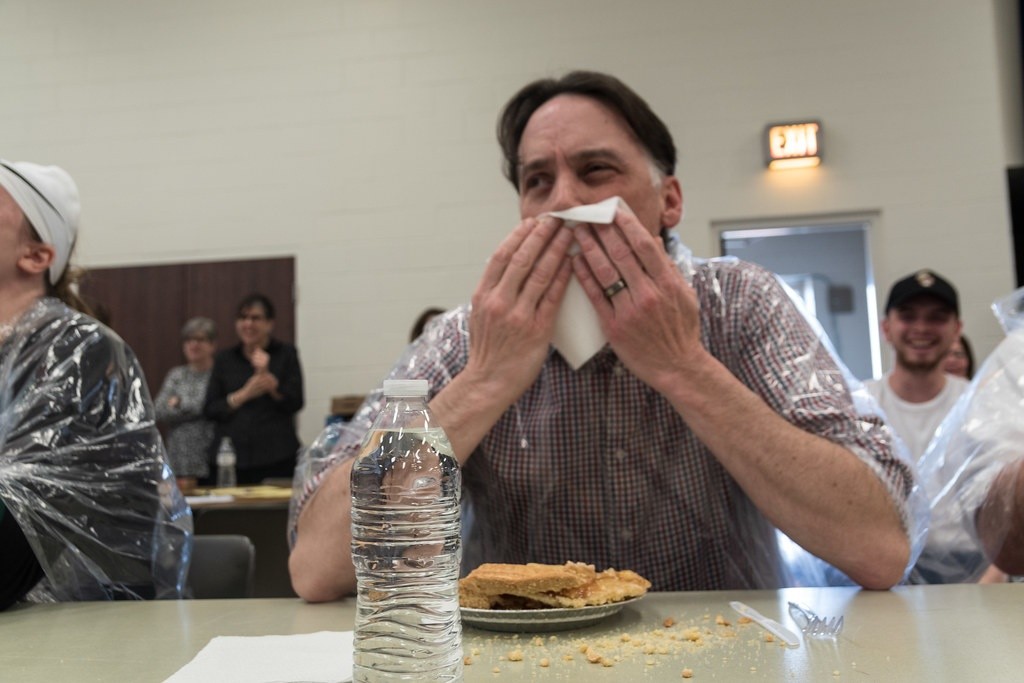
[216,436,238,488]
[350,380,463,683]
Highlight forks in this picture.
[784,601,847,637]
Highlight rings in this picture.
[606,280,625,297]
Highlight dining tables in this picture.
[0,582,1024,683]
[178,488,297,599]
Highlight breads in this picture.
[457,563,650,606]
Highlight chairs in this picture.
[192,535,257,598]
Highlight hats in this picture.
[884,270,958,317]
[0,159,80,284]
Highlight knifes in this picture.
[729,600,801,645]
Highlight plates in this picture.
[460,593,646,632]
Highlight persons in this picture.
[204,292,305,484]
[152,316,220,493]
[0,160,189,616]
[907,325,1024,587]
[858,270,971,470]
[409,307,446,344]
[286,70,914,604]
[937,331,973,380]
